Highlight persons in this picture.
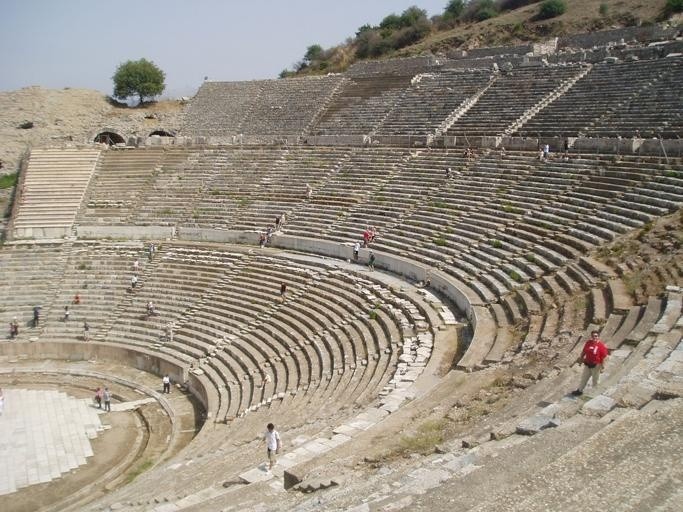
[571,330,608,396]
[538,148,544,162]
[263,424,280,470]
[103,387,110,412]
[305,184,313,200]
[163,374,170,394]
[563,140,571,156]
[280,281,286,303]
[543,142,550,160]
[10,244,173,342]
[95,387,102,408]
[354,226,377,273]
[259,212,286,249]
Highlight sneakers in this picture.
[572,390,582,395]
